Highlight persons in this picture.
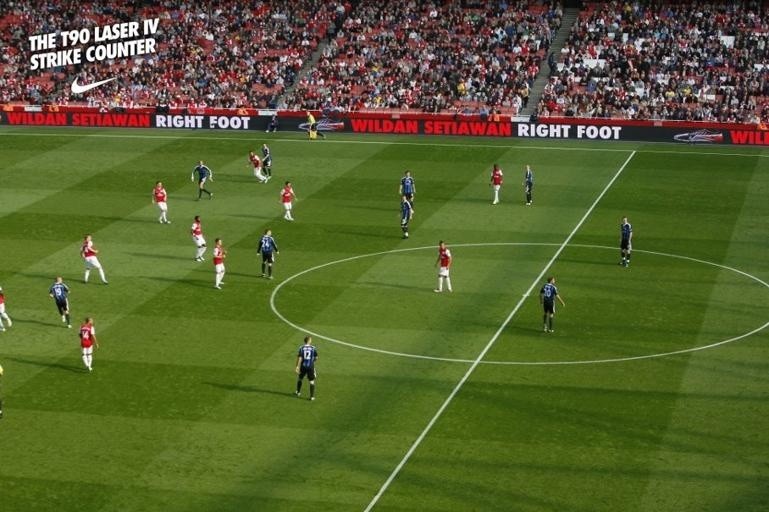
[212,238,228,289]
[522,165,533,205]
[539,277,565,333]
[79,234,109,285]
[489,163,503,204]
[432,241,452,293]
[1,1,769,139]
[293,335,318,401]
[190,215,207,262]
[399,196,412,239]
[248,151,268,183]
[256,229,280,279]
[0,286,12,331]
[48,276,73,329]
[78,317,99,371]
[190,160,213,201]
[278,181,298,222]
[618,216,633,268]
[261,143,272,180]
[152,182,171,224]
[398,171,416,210]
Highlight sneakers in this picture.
[6,318,12,328]
[220,282,224,285]
[209,192,213,200]
[200,256,205,261]
[434,289,442,293]
[214,286,222,290]
[295,391,300,397]
[192,257,201,262]
[61,315,65,322]
